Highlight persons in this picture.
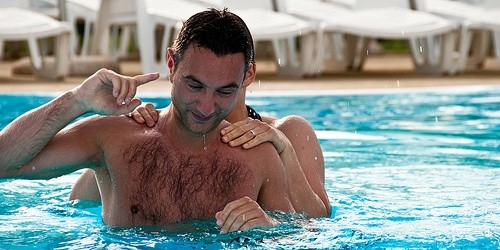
[68,12,331,220]
[0,6,298,235]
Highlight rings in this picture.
[250,130,256,137]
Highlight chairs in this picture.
[1,6,72,81]
[29,0,500,75]
